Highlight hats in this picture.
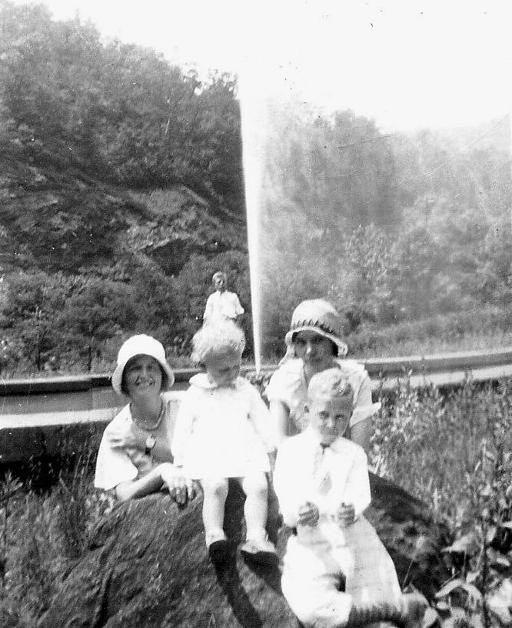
[283,299,348,357]
[109,333,174,397]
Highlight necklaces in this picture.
[131,396,168,450]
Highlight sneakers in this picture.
[204,529,278,567]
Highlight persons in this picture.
[168,318,281,565]
[269,367,428,627]
[202,270,246,326]
[90,331,196,507]
[262,295,382,465]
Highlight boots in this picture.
[346,591,429,627]
